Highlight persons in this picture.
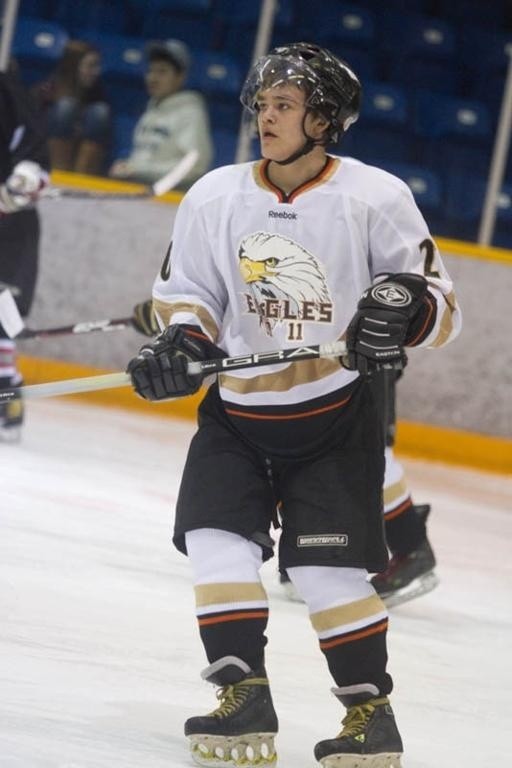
[131,295,440,616]
[109,37,213,191]
[123,43,464,768]
[2,68,48,442]
[26,38,109,172]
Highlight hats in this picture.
[140,37,191,69]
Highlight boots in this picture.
[369,499,436,594]
[0,373,26,427]
[314,681,404,759]
[183,656,280,738]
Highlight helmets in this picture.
[239,41,363,145]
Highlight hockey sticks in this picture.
[2,286,136,341]
[10,149,199,197]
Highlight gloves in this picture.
[126,325,218,403]
[344,273,437,383]
[0,161,47,215]
[132,300,162,338]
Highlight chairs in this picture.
[0,0,512,250]
